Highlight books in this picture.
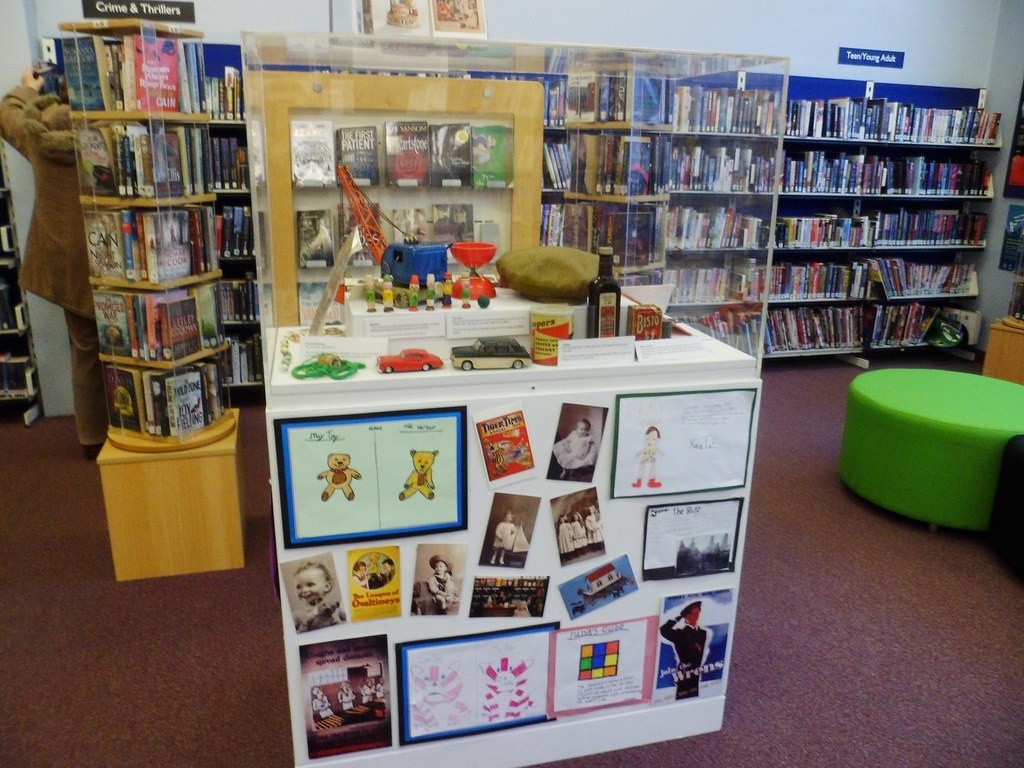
[536,74,1024,355]
[63,31,268,438]
[386,118,432,188]
[468,124,509,188]
[391,208,428,245]
[289,119,337,189]
[296,281,341,326]
[297,209,335,269]
[432,202,475,264]
[335,124,381,188]
[430,123,473,188]
[337,203,382,268]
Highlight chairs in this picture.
[837,365,1023,539]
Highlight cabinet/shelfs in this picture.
[1,47,996,392]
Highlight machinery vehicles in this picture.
[335,162,450,309]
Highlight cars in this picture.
[376,348,443,374]
[450,336,533,370]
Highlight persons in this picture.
[552,416,598,481]
[489,510,517,567]
[291,559,346,633]
[485,586,541,619]
[426,552,460,615]
[0,65,110,463]
[556,504,603,560]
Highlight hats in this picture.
[429,554,454,572]
[680,601,702,618]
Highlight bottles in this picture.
[586,246,622,340]
